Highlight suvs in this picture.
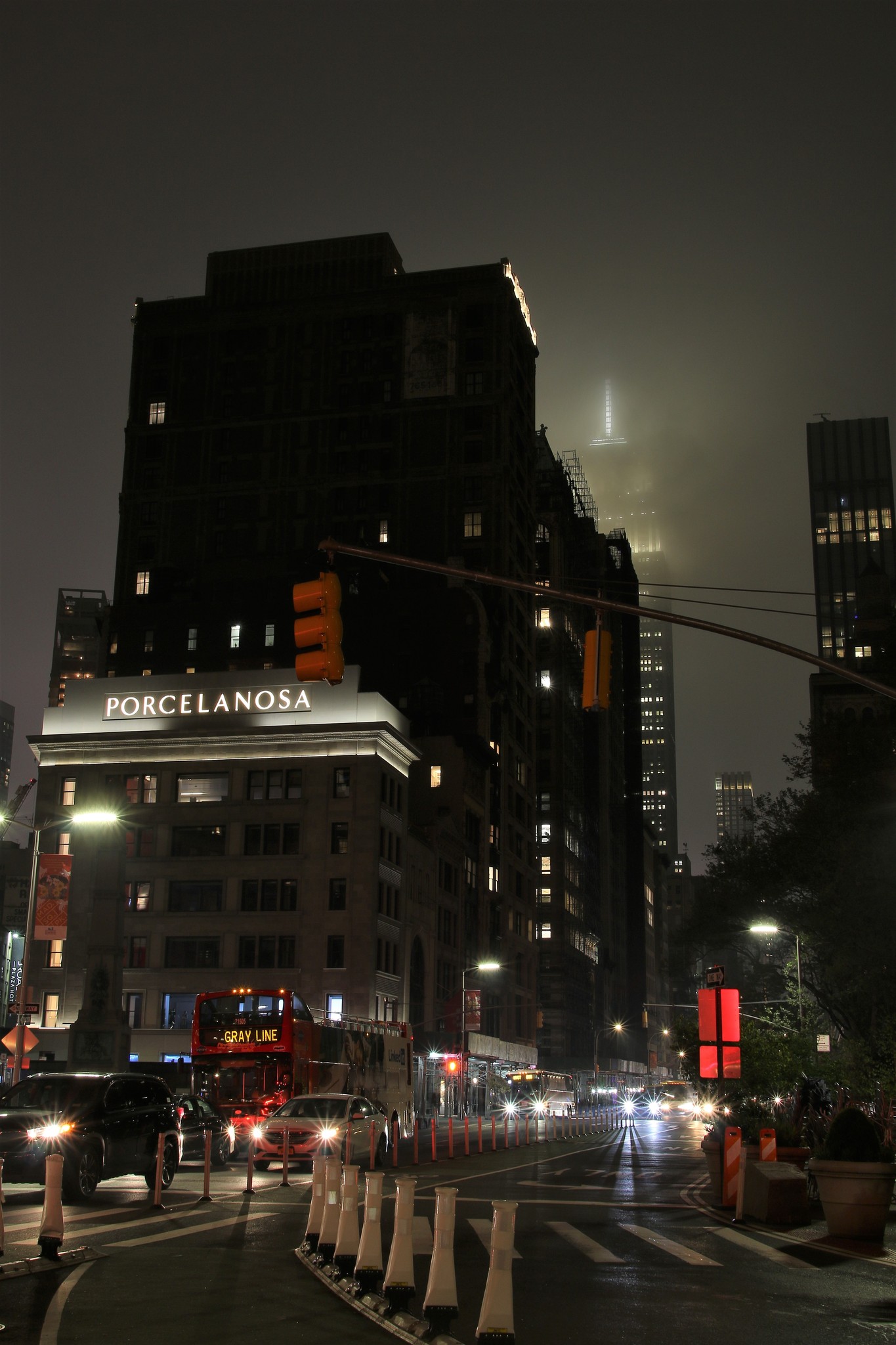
[1,1072,183,1198]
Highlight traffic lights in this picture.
[291,573,347,684]
[537,1011,543,1028]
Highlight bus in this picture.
[506,1069,577,1120]
[625,1081,700,1122]
[179,988,414,1161]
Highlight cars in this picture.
[252,1092,389,1169]
[172,1094,235,1166]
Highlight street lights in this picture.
[593,1023,684,1115]
[457,961,501,1120]
[0,811,120,1084]
[745,923,805,1029]
